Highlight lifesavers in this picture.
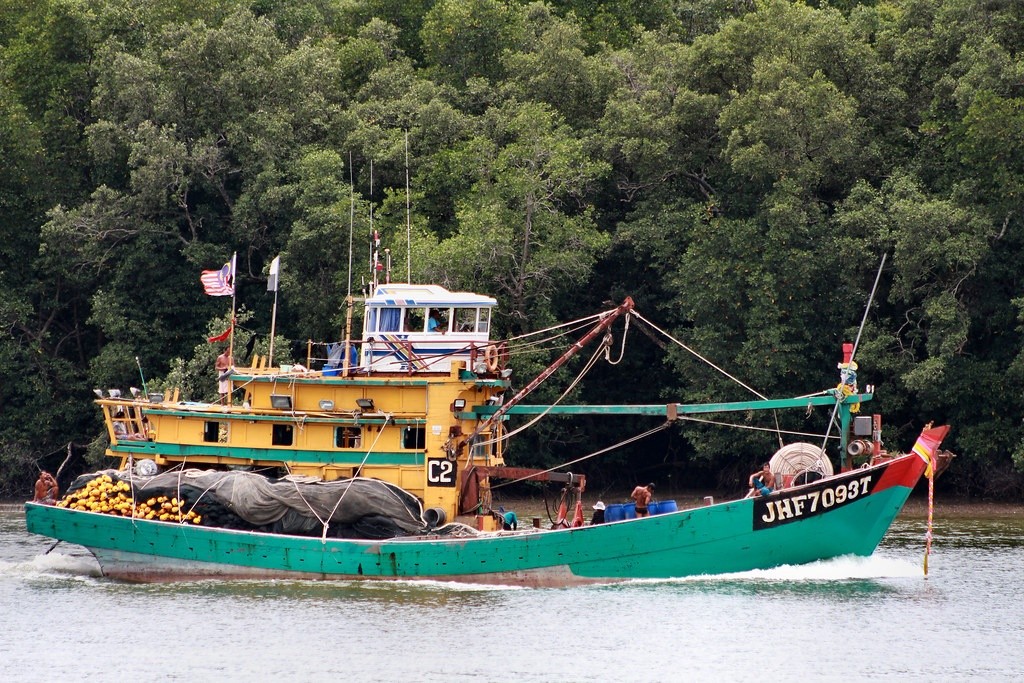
[484,345,499,372]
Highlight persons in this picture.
[33,471,58,506]
[749,463,774,496]
[631,483,655,518]
[215,346,234,406]
[404,309,446,334]
[589,501,605,525]
[113,411,145,440]
[502,512,517,530]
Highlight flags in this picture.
[201,255,236,296]
[268,256,279,291]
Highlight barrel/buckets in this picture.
[322,366,336,376]
[341,344,358,372]
[604,500,679,525]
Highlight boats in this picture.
[24,133,956,589]
